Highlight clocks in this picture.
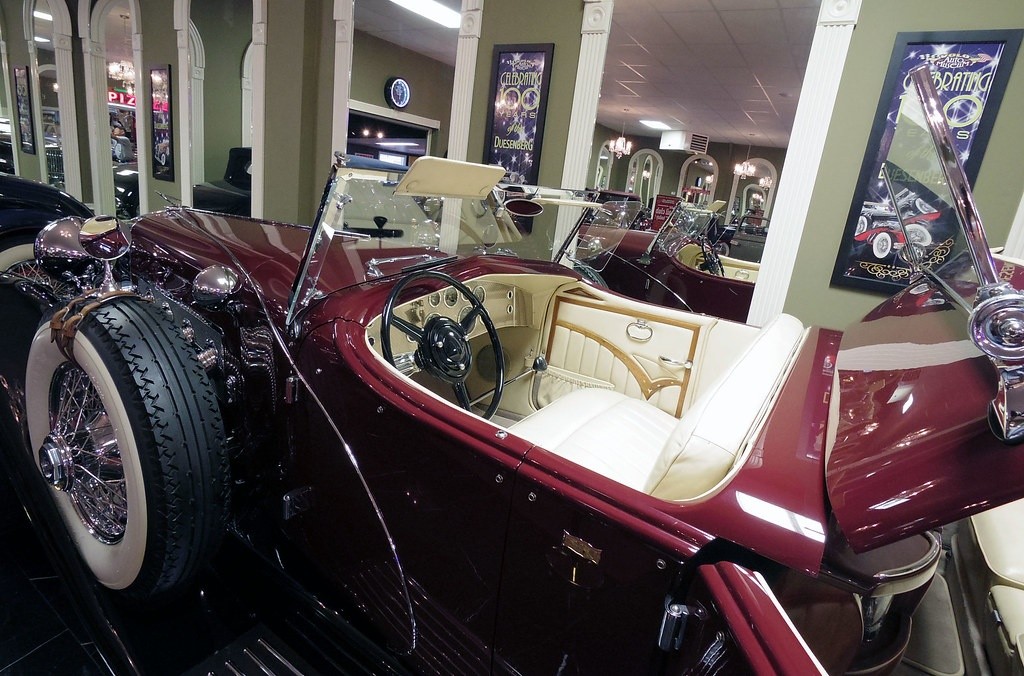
[384,77,411,109]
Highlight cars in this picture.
[0,154,1024,674]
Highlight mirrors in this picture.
[330,0,484,259]
[78,0,152,222]
[174,0,267,219]
[23,0,83,202]
[0,29,20,176]
[552,0,865,469]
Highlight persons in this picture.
[110,125,136,162]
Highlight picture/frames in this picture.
[13,64,36,155]
[828,28,1024,298]
[482,42,555,237]
[149,63,174,183]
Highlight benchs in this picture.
[507,312,805,501]
